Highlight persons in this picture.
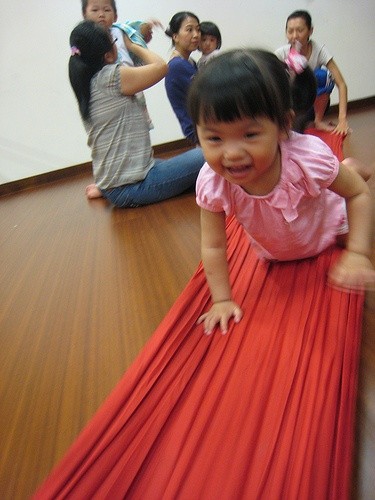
[186,45,375,338]
[66,18,205,209]
[195,22,223,67]
[81,0,155,131]
[274,9,355,138]
[164,10,202,148]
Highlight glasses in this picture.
[103,34,118,54]
